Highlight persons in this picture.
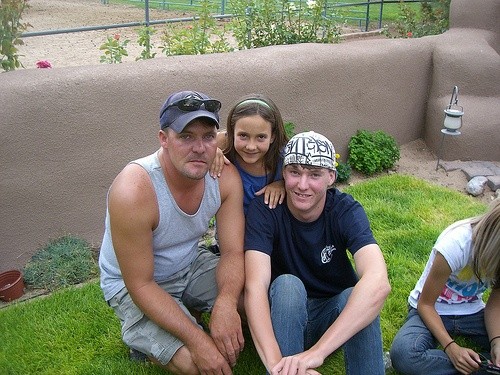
[98,90,389,375]
[390,197,500,375]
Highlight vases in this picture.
[0,268,23,303]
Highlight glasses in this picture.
[160,98,221,118]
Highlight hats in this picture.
[159,91,219,134]
[283,131,338,180]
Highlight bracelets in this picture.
[444,341,454,352]
[489,336,500,343]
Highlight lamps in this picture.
[435,85,463,169]
